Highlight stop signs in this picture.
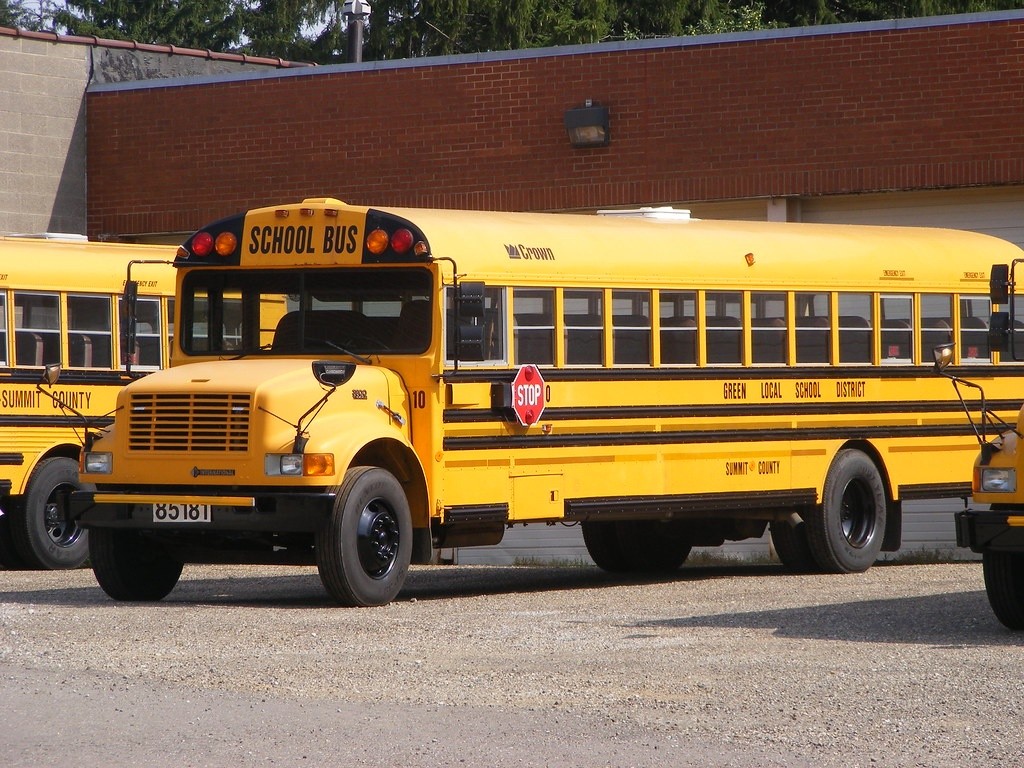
[512,364,545,426]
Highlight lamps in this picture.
[565,105,610,147]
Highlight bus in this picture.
[1,228,287,569]
[36,195,1024,610]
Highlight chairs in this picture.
[389,301,431,349]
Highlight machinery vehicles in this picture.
[932,258,1024,632]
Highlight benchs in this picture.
[0,327,232,367]
[275,300,989,363]
